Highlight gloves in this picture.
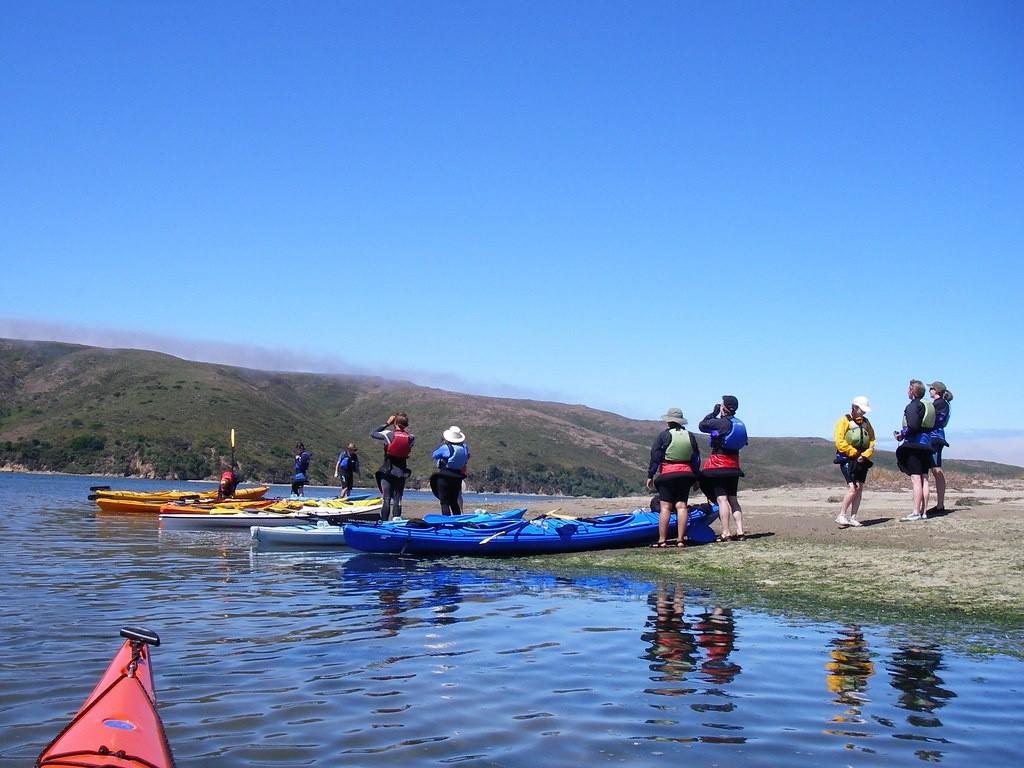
[713,404,721,416]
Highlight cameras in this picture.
[893,431,903,442]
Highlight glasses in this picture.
[930,388,934,390]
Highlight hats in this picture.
[443,426,465,443]
[927,381,946,394]
[722,395,738,412]
[660,407,687,425]
[344,443,359,452]
[294,440,306,451]
[852,396,871,413]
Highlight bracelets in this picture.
[386,423,389,426]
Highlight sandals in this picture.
[649,541,666,548]
[676,541,685,548]
[714,534,732,542]
[731,534,745,541]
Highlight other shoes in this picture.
[393,517,402,522]
[849,518,862,527]
[922,513,927,519]
[835,515,850,524]
[900,513,921,522]
[927,506,946,518]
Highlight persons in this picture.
[335,442,362,498]
[370,412,415,522]
[834,397,876,526]
[292,442,310,496]
[432,426,470,515]
[895,380,953,521]
[218,472,239,498]
[646,408,701,548]
[699,395,746,542]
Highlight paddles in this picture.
[479,506,561,544]
[230,429,235,472]
[269,507,327,520]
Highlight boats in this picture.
[86,483,383,527]
[340,501,721,556]
[249,507,527,547]
